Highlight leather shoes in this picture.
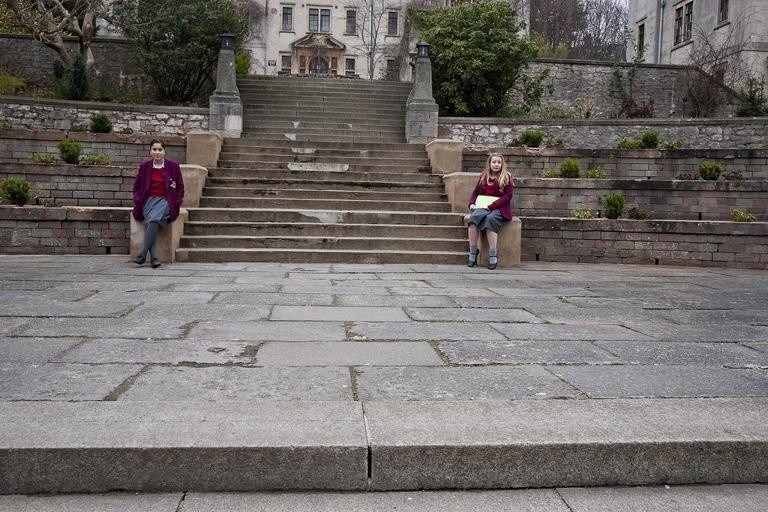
[468,249,479,267]
[488,253,498,269]
[133,256,144,265]
[152,260,161,268]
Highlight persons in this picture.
[465,152,514,270]
[132,139,185,268]
